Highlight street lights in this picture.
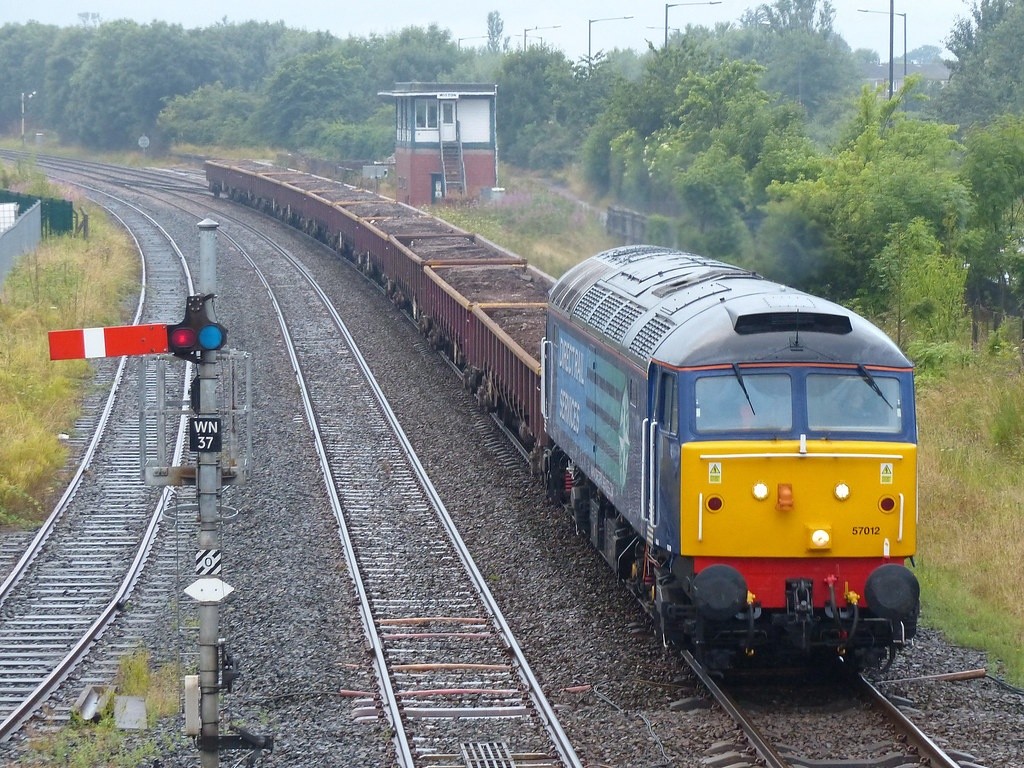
[458,36,489,51]
[665,2,722,47]
[524,25,562,54]
[858,8,907,85]
[515,34,542,49]
[588,16,632,70]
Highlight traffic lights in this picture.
[169,296,227,353]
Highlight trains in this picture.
[205,156,921,682]
